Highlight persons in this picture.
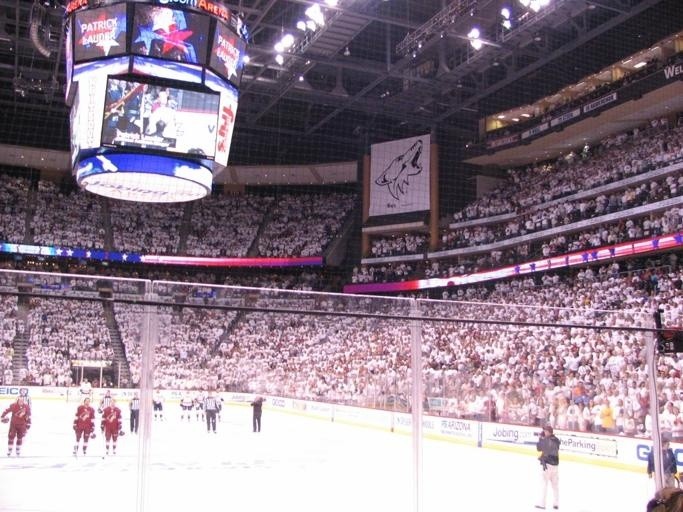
[647,438,677,490]
[0,398,30,455]
[72,396,95,455]
[534,425,560,509]
[100,399,123,455]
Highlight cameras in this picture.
[533,431,545,437]
[540,457,548,470]
